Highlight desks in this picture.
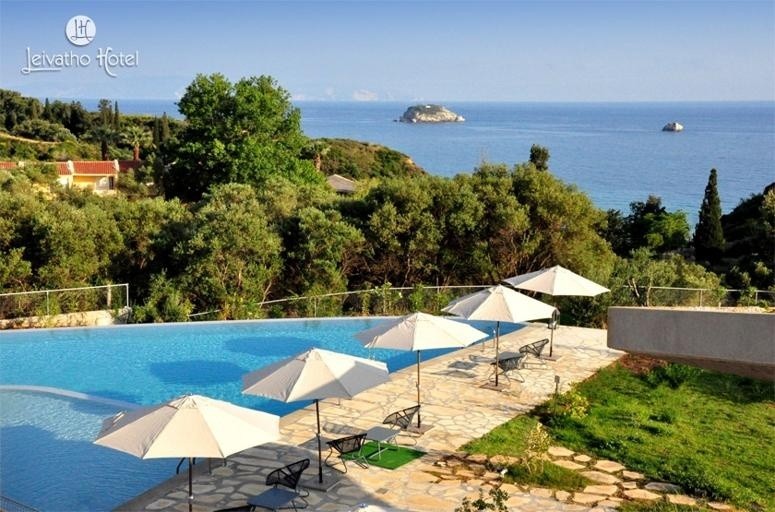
[246,487,299,511]
[495,351,522,359]
[358,426,400,463]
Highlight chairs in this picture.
[213,504,257,511]
[383,405,422,446]
[324,433,370,473]
[519,339,549,370]
[488,354,526,386]
[265,458,310,508]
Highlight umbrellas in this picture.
[440,284,557,388]
[353,311,489,427]
[92,392,280,512]
[501,265,609,357]
[242,347,391,483]
